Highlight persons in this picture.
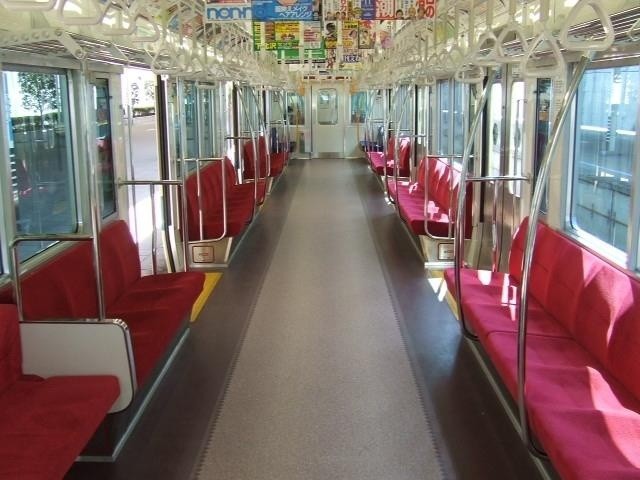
[340,12,347,20]
[394,9,404,21]
[405,7,418,20]
[348,11,355,20]
[417,7,427,19]
[325,23,337,42]
[325,12,334,20]
[334,11,341,20]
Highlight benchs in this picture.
[367,134,412,192]
[0,217,206,479]
[171,156,269,272]
[359,127,385,152]
[441,214,640,479]
[385,154,473,267]
[272,127,296,160]
[243,135,291,197]
